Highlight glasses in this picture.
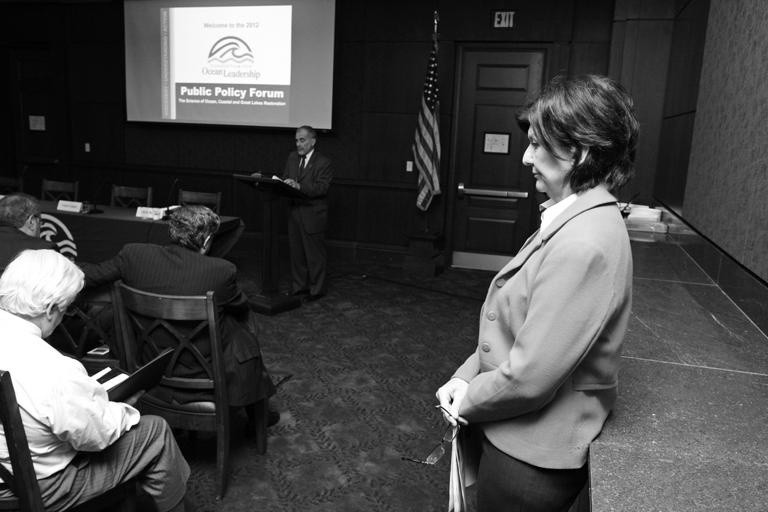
[401,405,461,466]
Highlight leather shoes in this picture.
[247,411,279,437]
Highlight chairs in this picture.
[177,189,222,212]
[111,184,152,210]
[113,278,266,499]
[2,177,79,201]
[1,370,138,512]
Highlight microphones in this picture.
[162,178,179,220]
[88,176,112,214]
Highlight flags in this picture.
[404,38,444,213]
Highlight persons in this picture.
[70,203,292,437]
[434,69,642,512]
[250,123,334,303]
[0,191,62,276]
[0,248,193,512]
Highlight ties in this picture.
[299,155,305,176]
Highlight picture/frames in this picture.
[482,131,511,156]
